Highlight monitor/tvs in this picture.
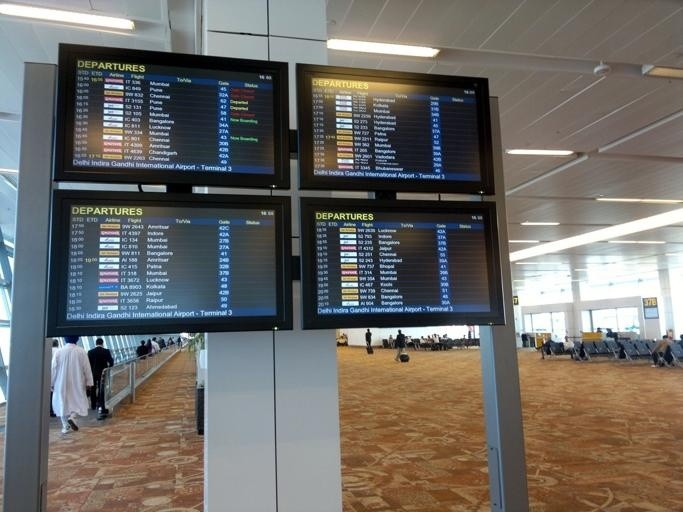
[299,196,507,331]
[53,42,291,192]
[44,189,294,338]
[295,62,496,196]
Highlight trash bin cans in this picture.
[197,380,204,436]
[383,340,389,349]
[522,335,536,348]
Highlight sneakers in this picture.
[68,418,79,430]
[63,429,69,433]
[394,359,399,362]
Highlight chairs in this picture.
[542,339,683,367]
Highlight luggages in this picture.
[366,342,373,354]
[400,348,410,362]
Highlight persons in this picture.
[133,333,182,374]
[387,328,453,351]
[606,327,619,342]
[49,338,59,416]
[86,337,115,410]
[650,334,672,368]
[49,335,94,435]
[563,335,578,359]
[364,328,372,346]
[677,334,683,350]
[542,337,555,356]
[393,329,405,362]
[336,332,348,347]
